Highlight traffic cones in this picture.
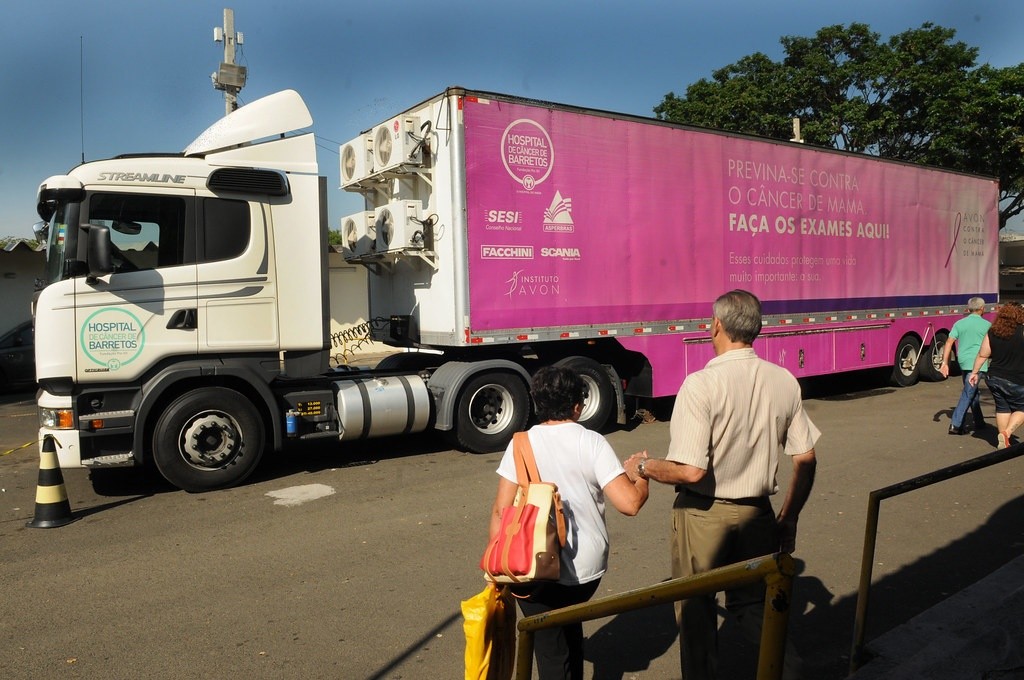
[27,433,81,529]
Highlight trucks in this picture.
[30,85,1001,495]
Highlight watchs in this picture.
[638,458,652,479]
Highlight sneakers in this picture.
[997,431,1010,450]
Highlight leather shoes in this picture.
[948,424,971,435]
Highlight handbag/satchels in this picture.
[460,585,515,680]
[480,432,566,584]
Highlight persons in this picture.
[488,366,650,680]
[624,289,822,679]
[968,301,1024,450]
[940,297,992,434]
